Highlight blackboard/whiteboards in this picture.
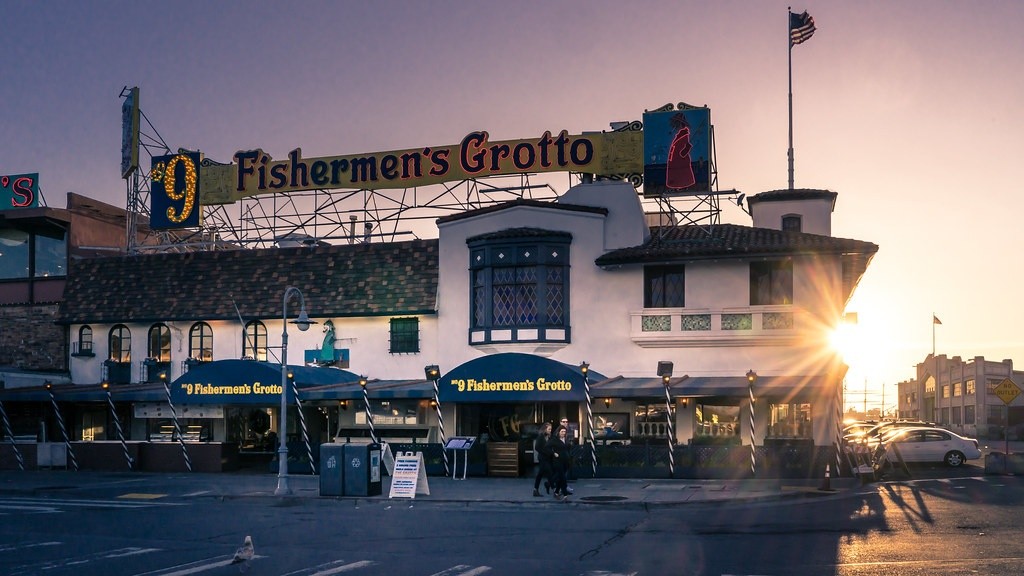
[389,451,422,499]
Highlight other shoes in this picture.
[544,482,551,494]
[564,491,572,496]
[560,486,573,491]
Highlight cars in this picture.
[841,417,942,447]
[852,427,982,468]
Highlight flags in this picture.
[790,10,817,47]
[934,316,942,324]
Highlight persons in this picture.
[320,319,336,363]
[252,430,277,450]
[611,415,624,433]
[532,416,574,503]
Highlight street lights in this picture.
[277,286,320,494]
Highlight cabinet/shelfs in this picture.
[486,440,524,478]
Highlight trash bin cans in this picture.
[320,442,382,497]
[984,451,1024,475]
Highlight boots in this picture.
[554,493,562,499]
[533,488,543,497]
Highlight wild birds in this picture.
[228,535,255,573]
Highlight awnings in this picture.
[299,377,436,402]
[169,359,361,406]
[0,382,171,403]
[588,374,837,400]
[437,351,609,404]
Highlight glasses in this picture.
[561,421,568,423]
